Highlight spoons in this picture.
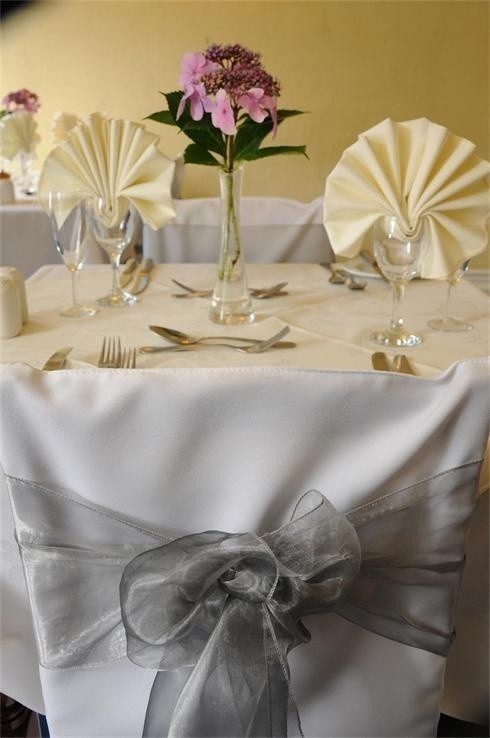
[171,278,288,299]
[148,324,297,349]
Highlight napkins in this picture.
[38,116,176,230]
[320,116,490,262]
[0,111,43,160]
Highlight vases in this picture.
[209,167,255,325]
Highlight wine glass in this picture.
[86,197,136,309]
[371,215,424,348]
[48,190,96,320]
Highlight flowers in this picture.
[143,39,309,161]
[2,88,42,115]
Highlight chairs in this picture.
[0,358,489,738]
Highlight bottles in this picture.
[0,276,23,339]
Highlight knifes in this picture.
[119,256,137,288]
[42,345,74,371]
[129,257,154,295]
[393,354,414,374]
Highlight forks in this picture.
[138,326,290,354]
[121,347,136,369]
[95,336,121,368]
